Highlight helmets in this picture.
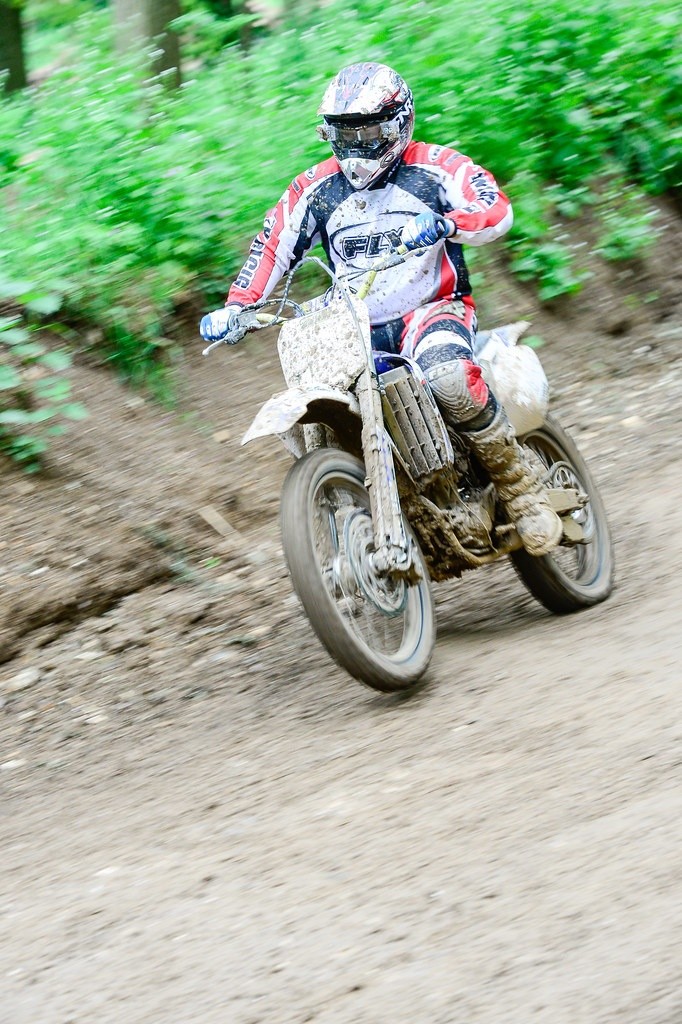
[316,61,416,191]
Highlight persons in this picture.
[199,63,564,557]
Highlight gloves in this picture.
[402,210,456,257]
[199,305,243,343]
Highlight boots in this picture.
[459,404,565,556]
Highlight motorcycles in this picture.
[203,219,615,697]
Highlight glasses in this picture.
[335,124,381,140]
